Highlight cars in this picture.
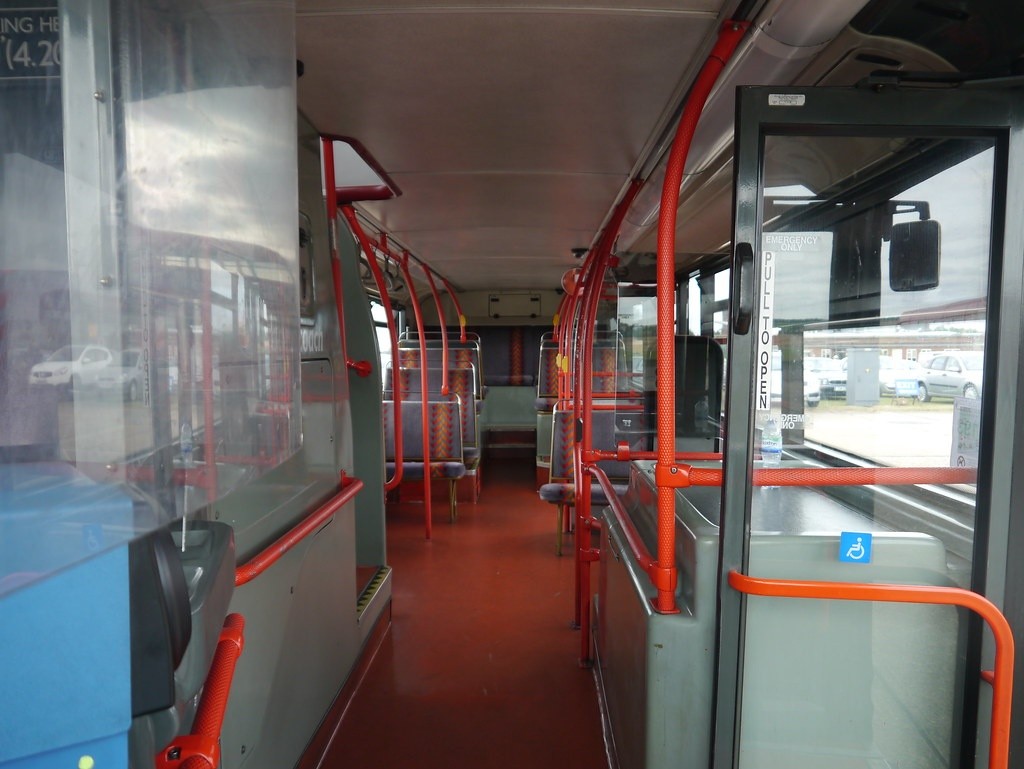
[631,355,644,391]
[768,347,919,407]
[29,343,221,405]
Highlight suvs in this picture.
[915,350,984,405]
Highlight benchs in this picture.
[383,334,654,558]
